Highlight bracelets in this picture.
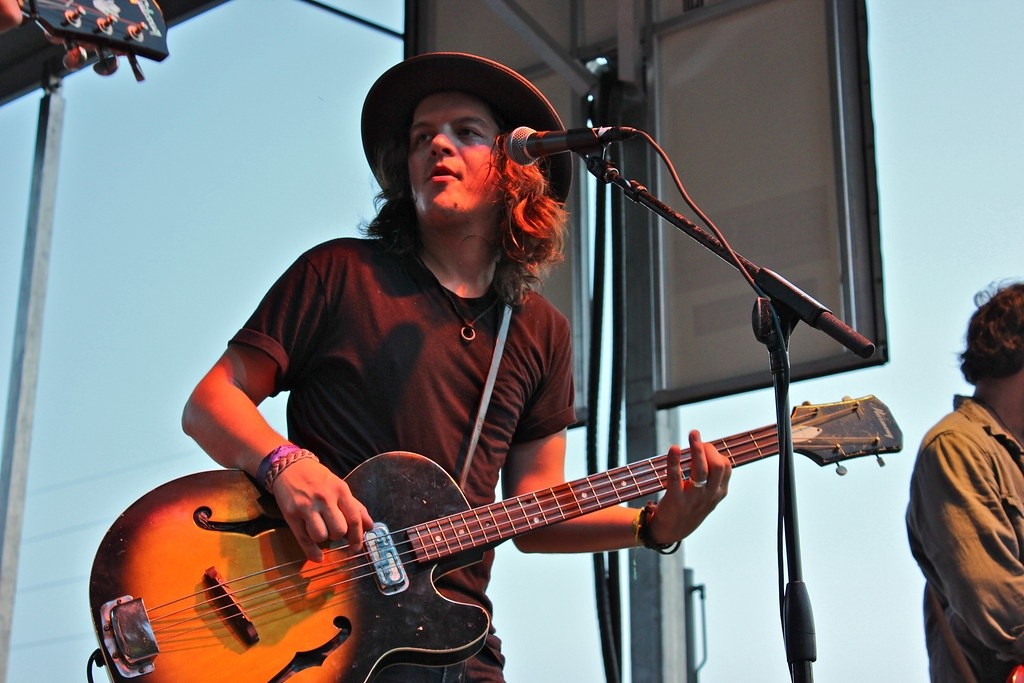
[264,449,320,493]
[637,499,682,554]
[256,444,301,481]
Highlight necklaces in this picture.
[413,252,501,343]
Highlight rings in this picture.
[688,477,707,488]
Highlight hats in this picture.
[361,52,573,208]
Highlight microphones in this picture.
[505,126,637,166]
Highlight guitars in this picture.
[89,394,903,683]
[0,0,170,82]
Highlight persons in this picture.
[181,51,731,683]
[905,284,1024,683]
